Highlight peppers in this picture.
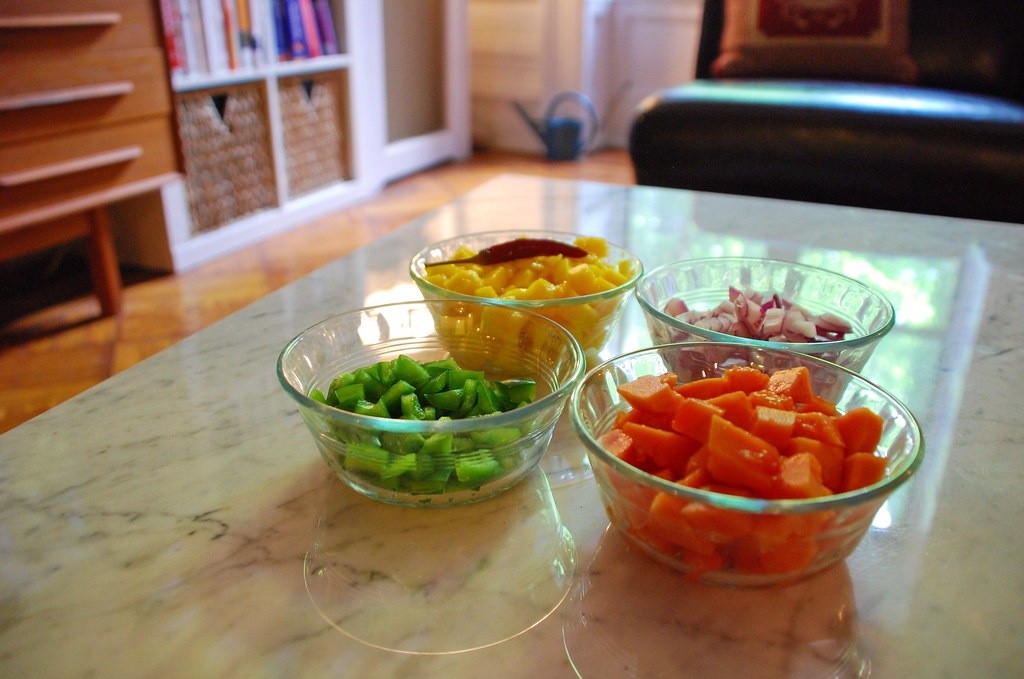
[306,354,541,495]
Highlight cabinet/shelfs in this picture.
[0,0,186,317]
[116,0,370,274]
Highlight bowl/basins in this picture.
[635,258,895,408]
[408,230,642,374]
[570,343,926,586]
[278,300,586,505]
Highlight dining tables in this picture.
[0,171,1022,679]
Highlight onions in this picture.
[662,285,856,404]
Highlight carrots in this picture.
[588,365,886,585]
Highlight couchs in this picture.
[629,0,1024,225]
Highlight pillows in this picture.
[710,0,918,85]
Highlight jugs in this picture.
[510,92,598,159]
